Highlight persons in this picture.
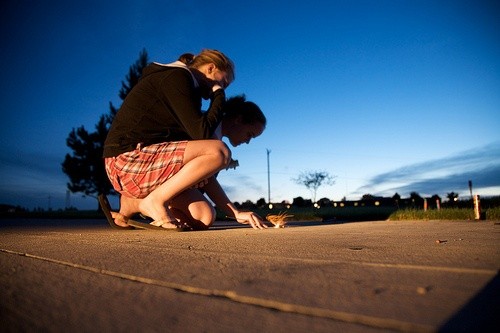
[103,49,235,230]
[201,96,268,231]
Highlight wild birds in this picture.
[265,209,295,228]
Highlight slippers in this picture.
[99,194,135,230]
[127,217,191,232]
